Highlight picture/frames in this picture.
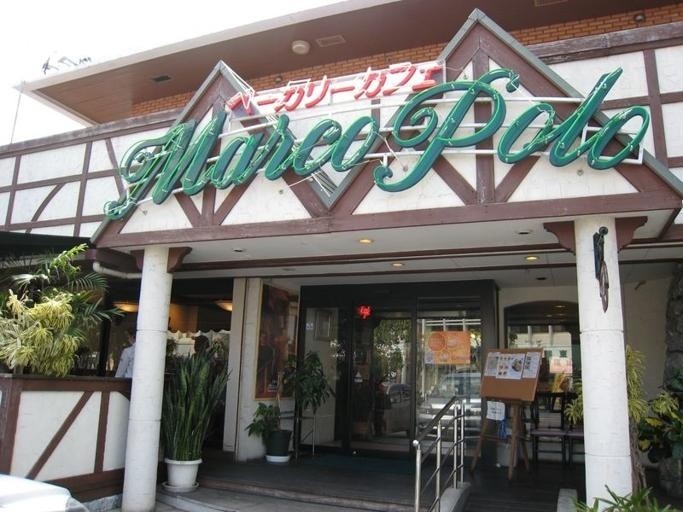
[313,309,336,342]
[253,278,301,402]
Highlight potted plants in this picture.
[244,402,294,464]
[162,340,231,492]
[275,351,320,461]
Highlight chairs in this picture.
[531,389,566,470]
[565,392,585,471]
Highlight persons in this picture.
[258,328,286,371]
[114,327,136,378]
